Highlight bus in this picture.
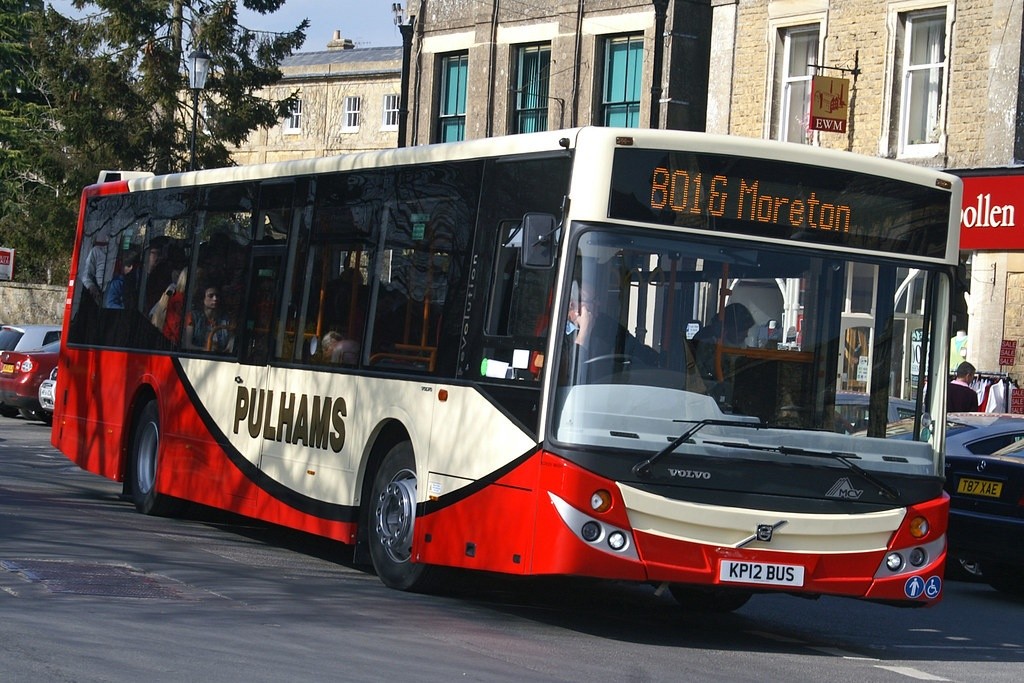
[47,126,967,612]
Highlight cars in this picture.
[852,410,1024,602]
[835,390,926,434]
[0,322,63,425]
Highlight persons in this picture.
[694,303,753,381]
[81,224,366,360]
[565,283,665,371]
[947,362,978,412]
[166,266,235,353]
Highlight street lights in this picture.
[389,0,422,147]
[188,40,213,171]
